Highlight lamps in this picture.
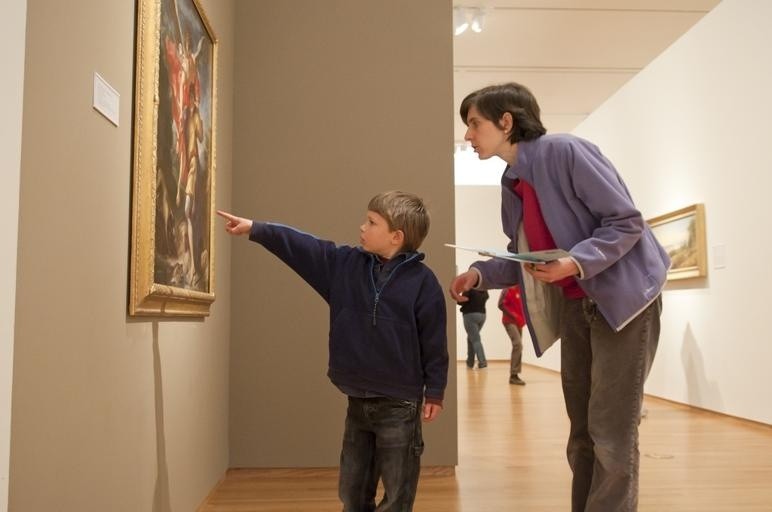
[453,6,489,35]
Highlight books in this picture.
[442,241,573,265]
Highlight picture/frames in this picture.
[127,0,220,319]
[644,202,708,283]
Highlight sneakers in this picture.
[510,376,525,385]
[466,361,486,368]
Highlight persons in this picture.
[458,289,490,369]
[177,83,206,278]
[450,82,672,511]
[217,192,450,512]
[499,283,530,385]
